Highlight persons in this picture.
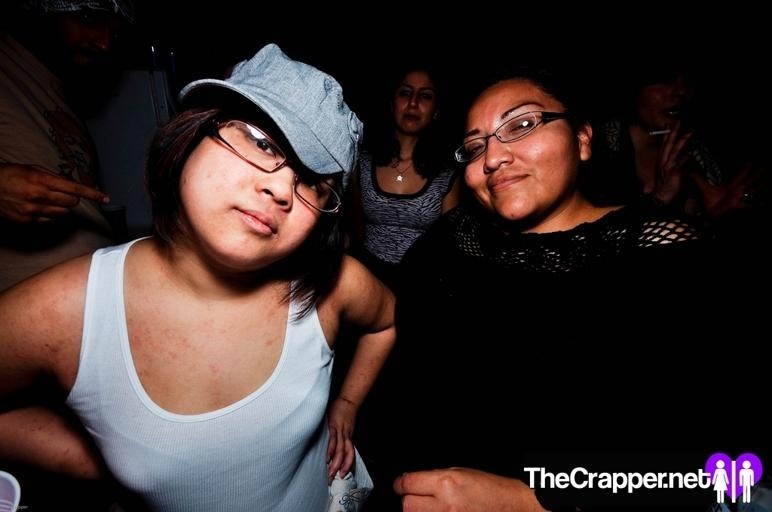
[0,1,771,511]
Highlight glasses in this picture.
[211,115,342,214]
[453,111,568,163]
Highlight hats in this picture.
[178,43,363,199]
